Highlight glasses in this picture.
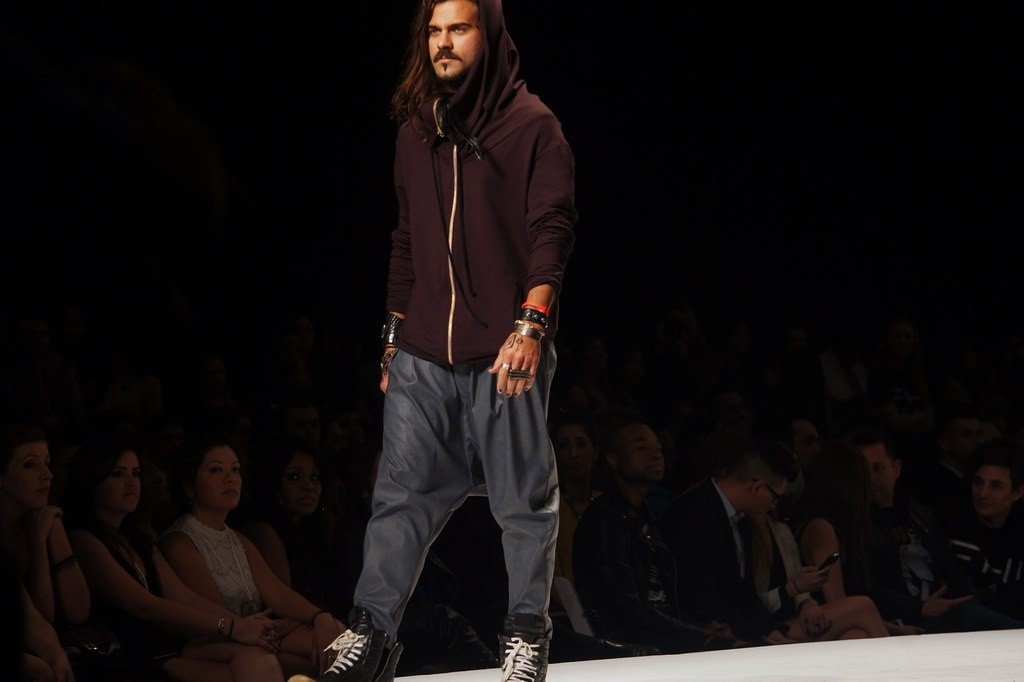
[750,477,783,507]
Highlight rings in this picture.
[821,629,825,633]
[329,656,335,658]
[265,635,271,641]
[502,362,510,370]
[509,369,531,380]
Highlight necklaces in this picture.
[120,534,150,592]
[188,525,254,606]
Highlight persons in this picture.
[316,0,580,682]
[551,292,1024,661]
[0,298,496,682]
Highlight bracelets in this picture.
[517,309,549,328]
[311,611,327,625]
[512,320,546,341]
[228,620,235,641]
[216,615,224,639]
[521,303,549,315]
[385,346,398,348]
[793,581,803,595]
[380,350,397,371]
[382,312,405,342]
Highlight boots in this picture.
[497,612,549,682]
[287,609,404,682]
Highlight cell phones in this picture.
[817,552,841,572]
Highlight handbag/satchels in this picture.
[51,625,127,682]
